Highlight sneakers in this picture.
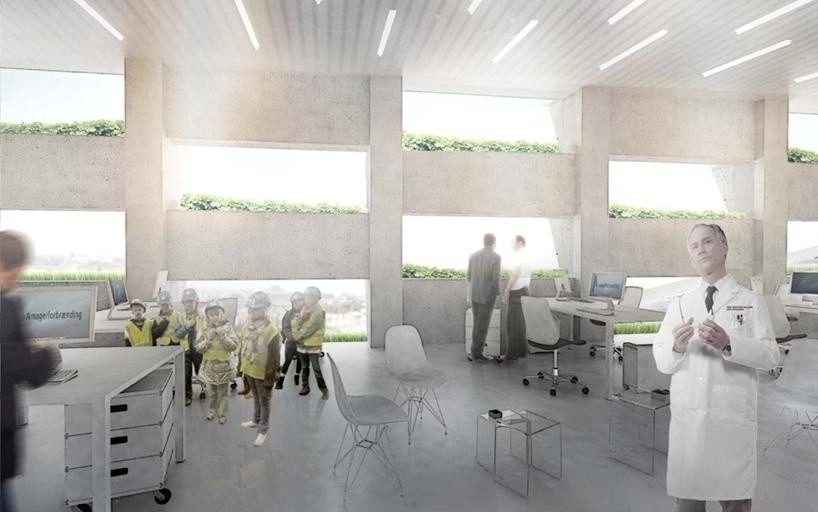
[471,354,531,364]
[254,433,267,447]
[205,413,229,425]
[241,421,258,429]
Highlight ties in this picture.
[702,286,718,313]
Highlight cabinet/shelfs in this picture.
[464,307,502,357]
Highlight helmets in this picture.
[289,291,304,302]
[180,288,199,306]
[244,291,272,310]
[304,286,322,301]
[129,298,147,314]
[157,290,174,306]
[203,299,226,318]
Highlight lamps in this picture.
[73,0,125,43]
[377,8,397,57]
[234,0,262,51]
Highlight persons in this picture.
[652,223,780,512]
[499,235,531,361]
[0,232,60,512]
[124,286,330,447]
[465,233,502,361]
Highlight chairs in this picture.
[590,285,643,362]
[764,294,808,378]
[762,406,817,452]
[326,324,449,509]
[519,295,590,396]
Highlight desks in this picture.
[541,295,666,402]
[12,344,186,511]
[474,407,564,500]
[60,302,162,347]
[603,388,671,477]
[784,300,818,314]
